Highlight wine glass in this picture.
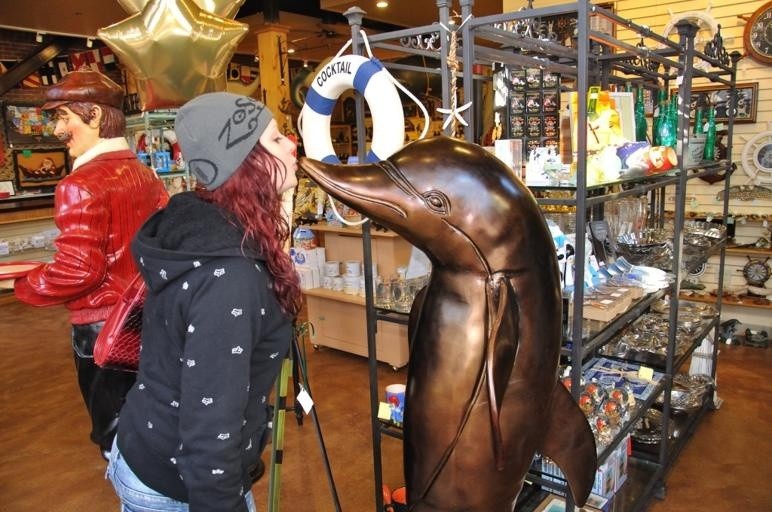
[600,197,648,246]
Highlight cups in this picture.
[384,382,407,426]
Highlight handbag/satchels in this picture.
[93,295,145,372]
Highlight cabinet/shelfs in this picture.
[677,242,772,355]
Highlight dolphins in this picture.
[299,135,597,512]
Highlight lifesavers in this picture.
[302,54,405,165]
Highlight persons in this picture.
[0,68,168,462]
[103,91,311,511]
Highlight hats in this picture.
[174,90,273,192]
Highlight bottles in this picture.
[692,105,717,161]
[633,87,679,150]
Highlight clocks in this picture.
[736,1,772,69]
[730,121,772,194]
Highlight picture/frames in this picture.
[665,82,758,124]
